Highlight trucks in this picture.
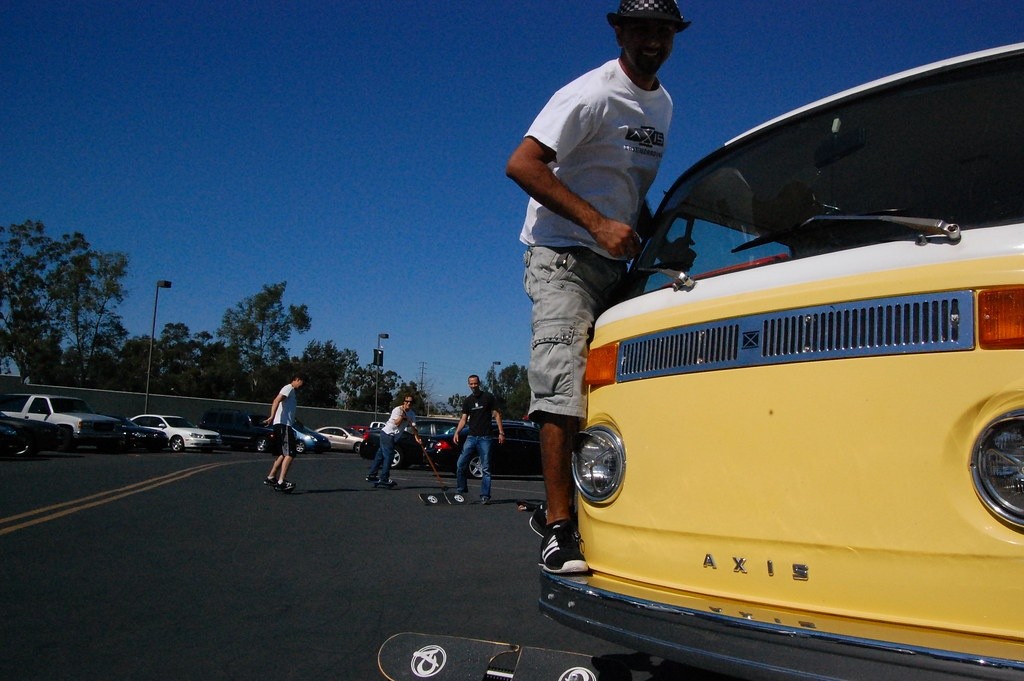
[538,40,1023,681]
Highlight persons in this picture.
[452,375,506,504]
[366,394,422,485]
[262,372,309,488]
[506,0,693,575]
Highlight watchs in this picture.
[499,433,505,435]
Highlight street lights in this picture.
[492,362,501,394]
[375,334,389,422]
[144,281,172,414]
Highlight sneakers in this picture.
[264,477,278,487]
[274,479,296,491]
[538,519,589,574]
[529,507,579,539]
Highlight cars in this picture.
[361,418,470,470]
[104,415,169,452]
[130,414,223,453]
[369,422,386,428]
[422,423,543,480]
[314,426,367,454]
[292,424,332,454]
[0,411,72,458]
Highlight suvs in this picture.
[199,407,297,453]
[0,394,125,454]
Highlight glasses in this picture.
[405,400,413,404]
[623,24,675,40]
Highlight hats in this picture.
[606,0,691,33]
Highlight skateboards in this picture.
[378,631,633,681]
[417,492,472,506]
[367,480,398,489]
[273,485,296,494]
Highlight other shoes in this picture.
[455,489,469,496]
[481,496,490,504]
[375,479,400,490]
[366,474,380,483]
